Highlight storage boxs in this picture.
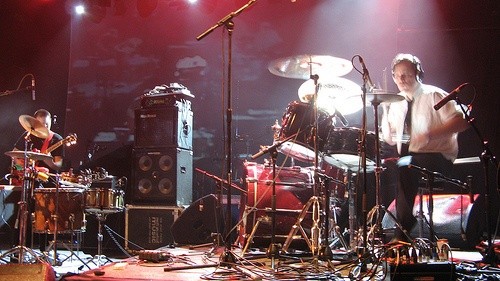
[124,204,188,254]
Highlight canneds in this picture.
[437,239,448,260]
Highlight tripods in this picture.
[162,0,267,281]
[235,71,414,281]
[0,129,113,274]
[453,95,500,266]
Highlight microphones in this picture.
[359,55,374,90]
[31,77,36,101]
[332,106,348,127]
[434,83,470,110]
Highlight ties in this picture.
[401,95,415,155]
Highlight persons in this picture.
[13,108,64,187]
[367,53,470,242]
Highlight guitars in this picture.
[43,133,77,154]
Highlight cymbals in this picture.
[349,91,407,106]
[19,114,49,138]
[267,55,354,77]
[298,77,367,114]
[5,150,54,160]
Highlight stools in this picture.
[410,181,444,254]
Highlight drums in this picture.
[327,126,384,173]
[277,100,333,161]
[33,187,86,234]
[84,185,126,213]
[237,160,327,252]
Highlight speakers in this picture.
[0,262,56,280]
[168,193,242,245]
[133,107,193,150]
[379,193,487,251]
[132,147,193,206]
